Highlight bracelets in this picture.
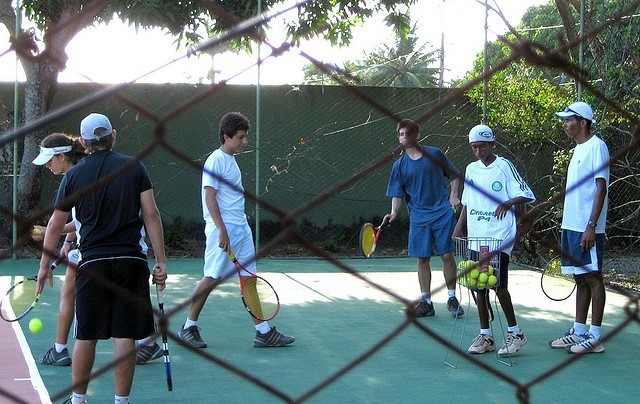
[63,240,73,245]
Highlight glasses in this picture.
[565,106,584,120]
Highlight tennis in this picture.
[31,229,43,240]
[29,318,43,334]
[457,260,497,290]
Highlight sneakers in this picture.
[134,341,165,364]
[499,329,527,355]
[566,334,605,352]
[469,332,496,352]
[446,297,464,316]
[63,397,87,404]
[178,325,207,348]
[407,300,436,317]
[550,328,590,348]
[254,326,294,347]
[37,345,71,366]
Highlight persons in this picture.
[30,132,166,367]
[450,124,537,356]
[549,101,611,354]
[381,120,465,317]
[33,113,168,403]
[177,110,296,348]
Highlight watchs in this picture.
[588,221,597,228]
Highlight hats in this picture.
[32,145,72,166]
[469,125,496,142]
[81,113,113,139]
[555,101,593,120]
